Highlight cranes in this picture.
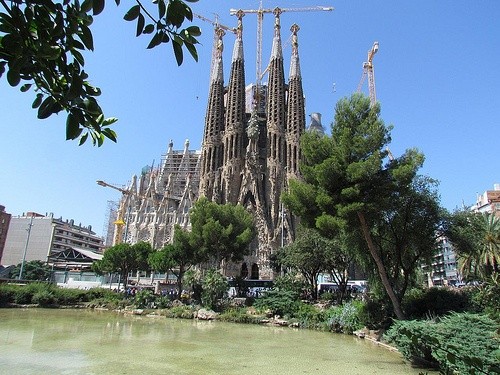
[230,0,335,110]
[356,41,380,108]
[95,180,167,247]
[190,8,233,81]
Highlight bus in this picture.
[315,280,368,296]
[222,276,280,298]
[432,276,476,289]
